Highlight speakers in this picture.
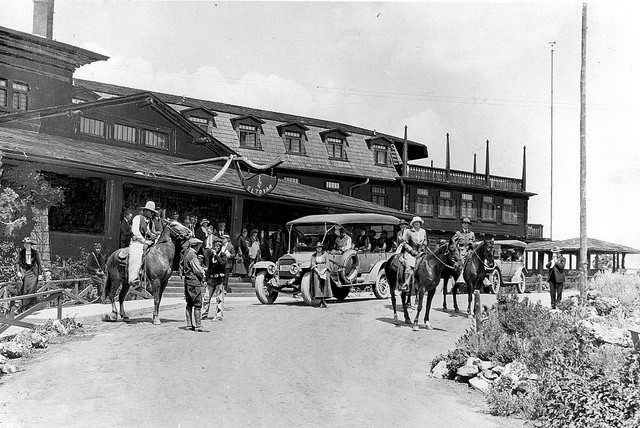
[195,308,210,331]
[186,306,195,329]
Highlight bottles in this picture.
[550,246,563,252]
[242,227,247,232]
[21,237,38,245]
[398,219,408,225]
[313,241,325,247]
[200,218,210,225]
[460,217,472,224]
[410,216,424,226]
[141,200,159,213]
[252,229,258,232]
[190,238,204,245]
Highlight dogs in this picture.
[103,216,194,325]
[440,237,496,319]
[385,238,464,332]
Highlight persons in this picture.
[396,219,409,246]
[85,242,107,301]
[546,246,566,308]
[195,218,210,265]
[128,200,159,285]
[201,241,227,320]
[369,230,377,251]
[268,227,284,262]
[237,228,252,278]
[329,229,352,254]
[248,229,262,277]
[310,241,333,306]
[500,245,511,262]
[170,210,183,271]
[511,247,523,262]
[183,238,211,332]
[388,225,401,249]
[185,215,197,231]
[213,223,235,293]
[403,216,428,291]
[376,229,389,250]
[354,228,369,250]
[152,207,165,232]
[120,210,132,248]
[14,237,42,308]
[451,217,492,287]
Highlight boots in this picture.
[253,213,401,305]
[476,240,527,294]
